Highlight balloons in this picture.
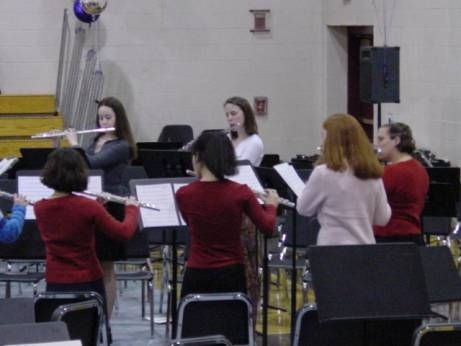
[73,0,108,23]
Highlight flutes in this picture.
[182,124,242,145]
[187,168,298,209]
[1,188,37,210]
[74,185,163,214]
[30,126,117,140]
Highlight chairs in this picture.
[158,125,194,143]
[1,206,461,346]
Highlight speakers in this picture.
[359,46,400,104]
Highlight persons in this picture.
[63,98,151,318]
[33,149,139,314]
[176,130,279,297]
[224,97,264,167]
[297,112,391,245]
[0,190,31,245]
[372,123,428,247]
